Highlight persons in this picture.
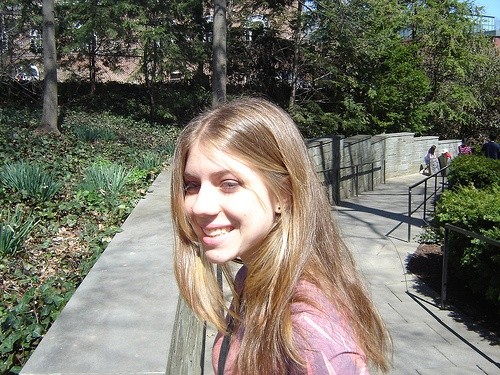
[420,132,500,175]
[170,98,395,375]
